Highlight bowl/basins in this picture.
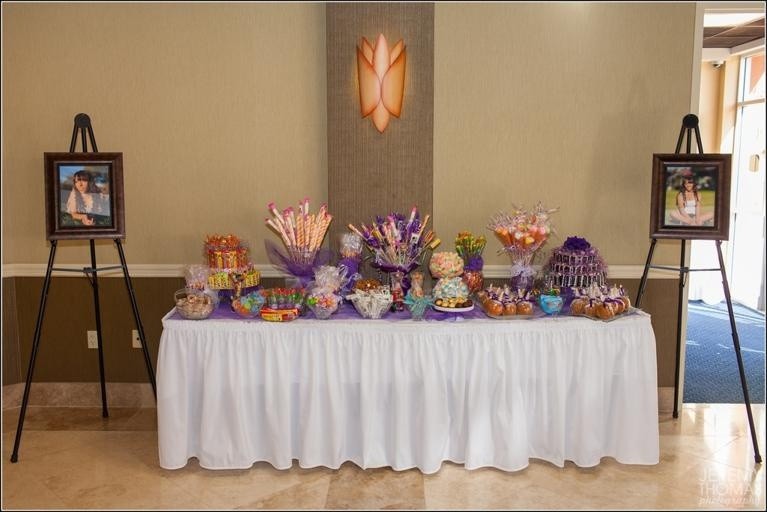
[228,289,395,320]
[174,298,216,321]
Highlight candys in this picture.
[175,197,630,320]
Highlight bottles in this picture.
[390,271,404,311]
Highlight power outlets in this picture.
[88,330,98,349]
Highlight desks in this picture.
[161,302,651,468]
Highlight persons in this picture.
[670,176,716,226]
[66,168,111,227]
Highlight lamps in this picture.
[356,31,407,134]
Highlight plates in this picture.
[471,285,546,320]
[430,302,474,313]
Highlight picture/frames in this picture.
[43,152,127,240]
[649,153,731,238]
